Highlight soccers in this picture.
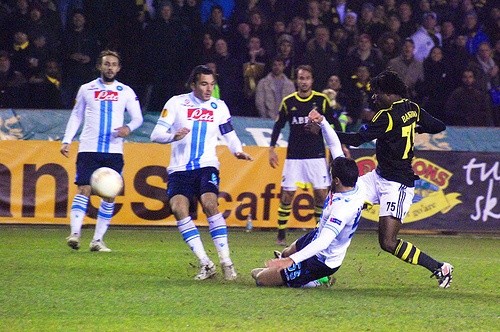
[90,166,123,199]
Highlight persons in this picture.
[250,109,366,289]
[269,65,351,246]
[0,0,500,127]
[60,50,143,252]
[151,66,255,280]
[305,70,454,288]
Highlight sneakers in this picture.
[89,240,112,252]
[430,261,453,289]
[273,250,281,258]
[324,274,336,288]
[221,262,237,281]
[66,233,80,250]
[195,263,217,281]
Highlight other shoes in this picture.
[276,239,286,245]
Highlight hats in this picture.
[277,34,293,55]
